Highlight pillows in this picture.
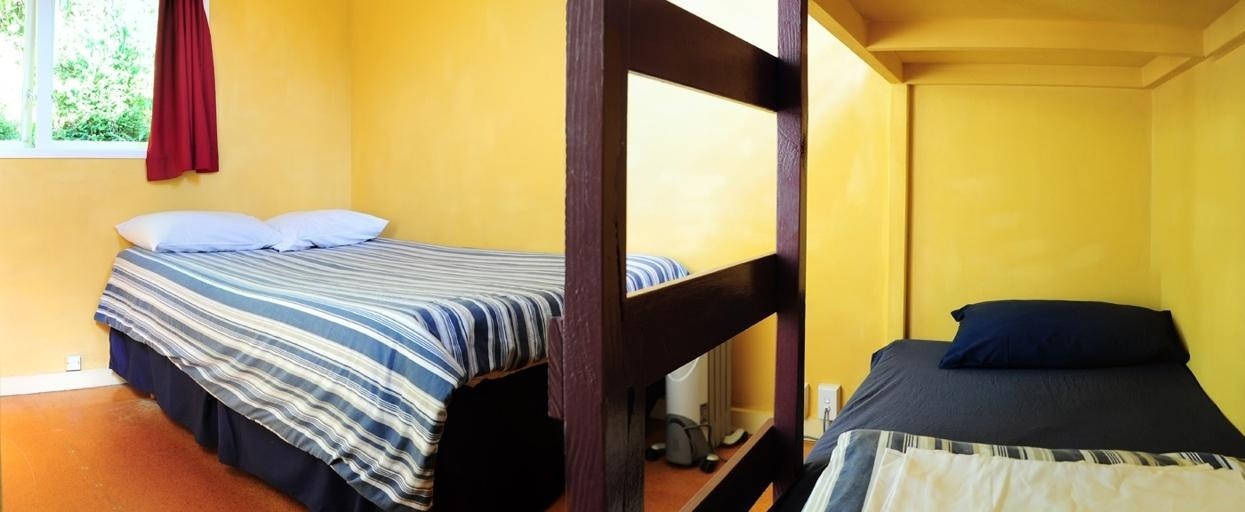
[264,211,391,252]
[116,211,281,253]
[941,300,1189,379]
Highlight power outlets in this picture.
[818,384,843,422]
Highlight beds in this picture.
[563,1,1244,511]
[94,205,691,509]
